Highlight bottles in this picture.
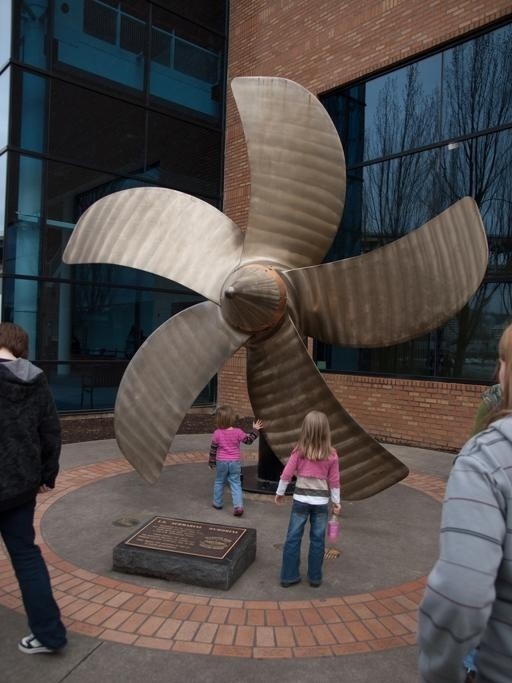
[328,513,340,543]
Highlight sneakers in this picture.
[309,579,322,587]
[212,503,223,509]
[233,506,243,516]
[18,633,59,654]
[281,577,301,587]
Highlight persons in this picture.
[0,322,67,656]
[274,410,341,587]
[417,324,512,683]
[470,384,502,439]
[208,406,263,516]
